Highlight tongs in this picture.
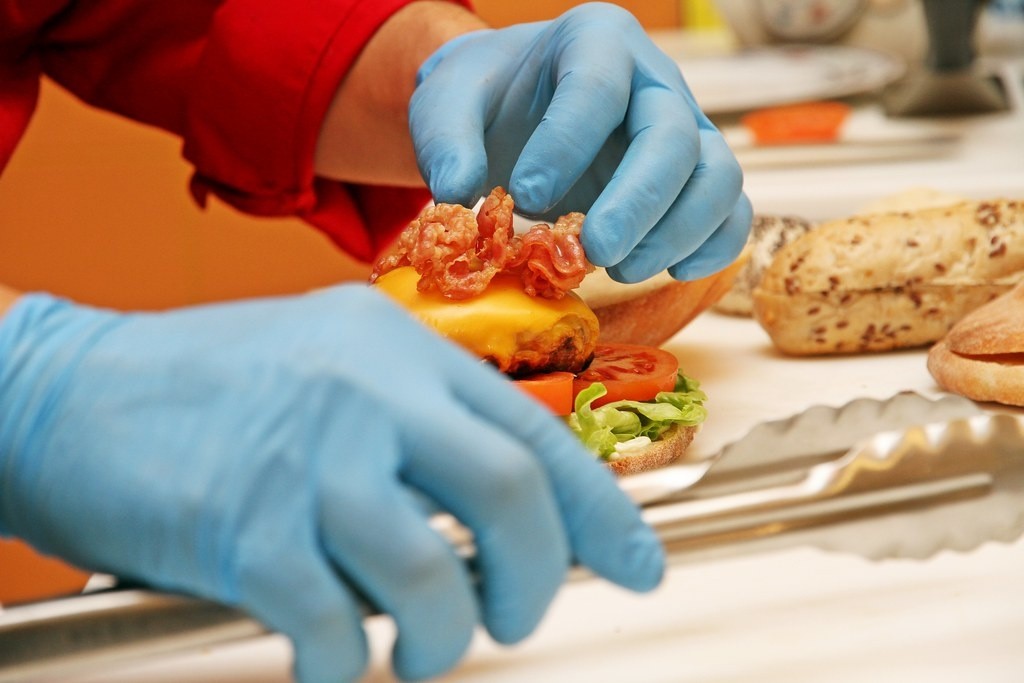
[0,388,1024,683]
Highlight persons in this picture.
[0,0,752,683]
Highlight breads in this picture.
[706,194,1023,410]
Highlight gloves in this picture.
[406,2,754,285]
[0,282,665,683]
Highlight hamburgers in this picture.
[365,186,753,487]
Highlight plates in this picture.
[678,45,903,114]
[2,423,716,628]
